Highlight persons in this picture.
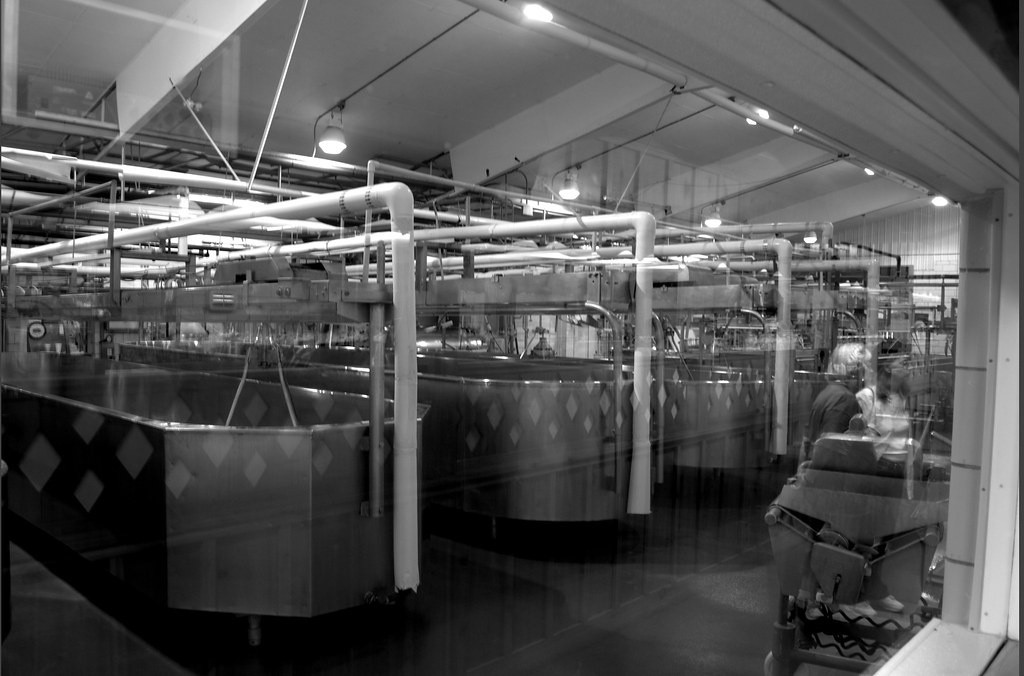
[798,343,877,615]
[843,337,922,616]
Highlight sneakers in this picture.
[843,601,877,617]
[877,595,904,612]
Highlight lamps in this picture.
[558,165,582,201]
[704,201,724,228]
[318,102,348,154]
